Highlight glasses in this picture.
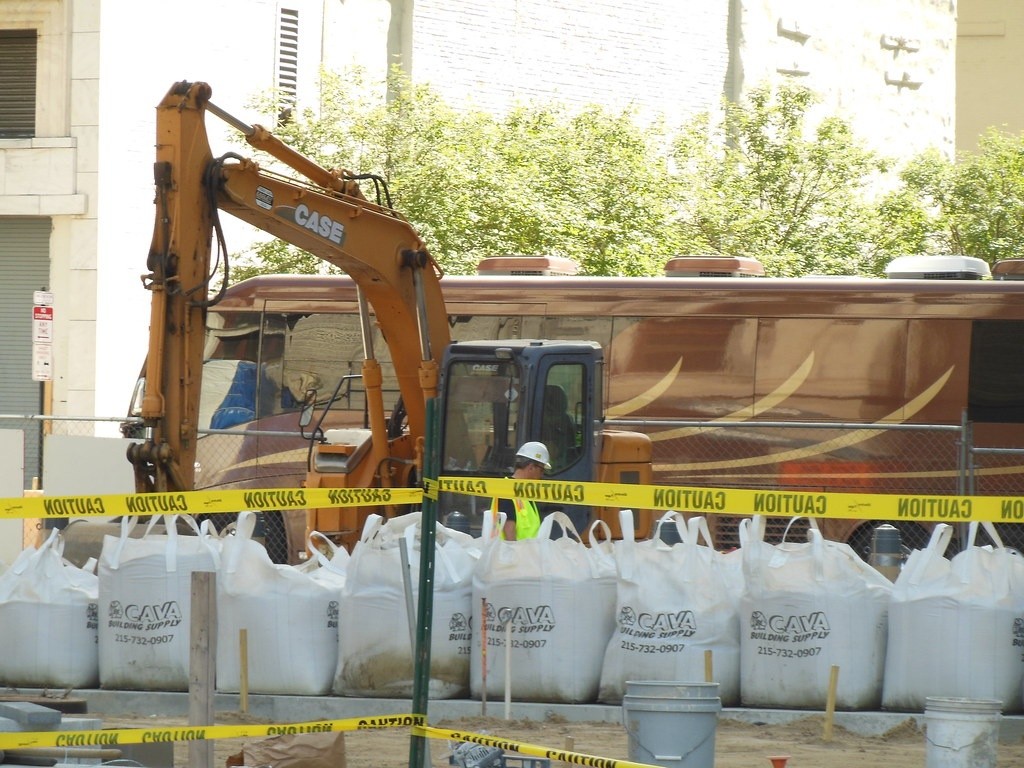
[535,463,548,474]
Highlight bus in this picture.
[119,250,1024,581]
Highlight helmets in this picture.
[516,441,552,470]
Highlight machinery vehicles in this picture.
[48,79,655,565]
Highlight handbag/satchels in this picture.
[0,507,1024,714]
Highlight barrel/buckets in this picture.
[919,696,1004,768]
[621,681,722,768]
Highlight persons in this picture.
[494,442,554,542]
